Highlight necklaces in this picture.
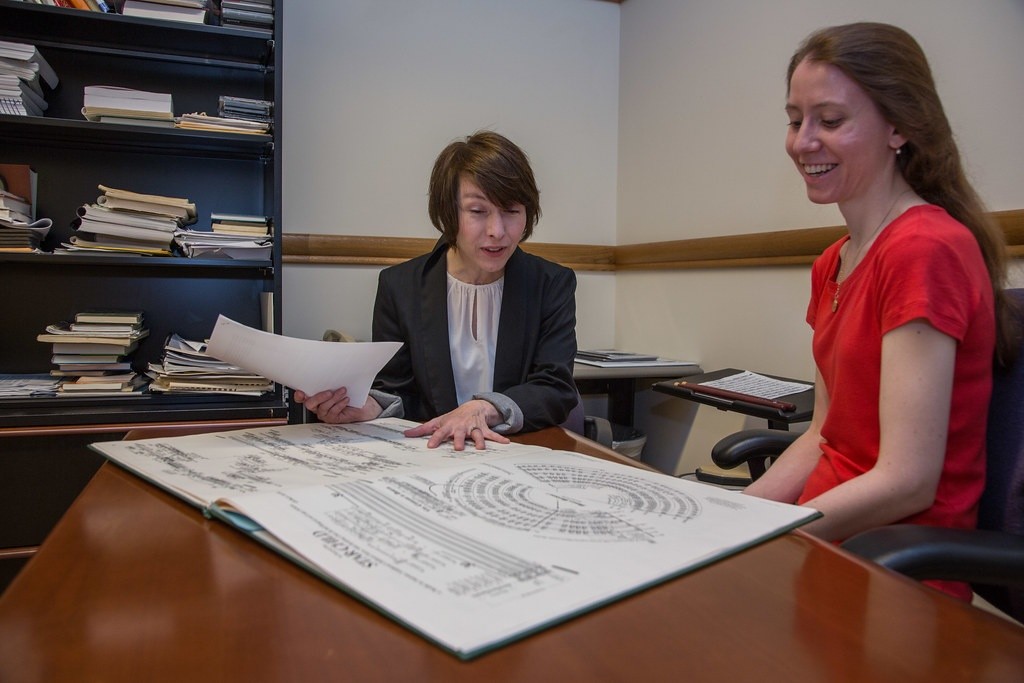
[832,186,912,313]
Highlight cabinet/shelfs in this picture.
[0,0,291,427]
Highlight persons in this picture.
[741,23,1011,608]
[293,130,578,450]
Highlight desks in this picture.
[573,358,705,476]
[0,415,1024,683]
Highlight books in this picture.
[145,332,276,398]
[10,0,276,36]
[81,85,276,138]
[54,184,275,261]
[37,311,150,396]
[88,418,824,661]
[0,42,58,117]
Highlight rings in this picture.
[470,427,483,438]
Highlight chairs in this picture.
[694,289,1024,623]
